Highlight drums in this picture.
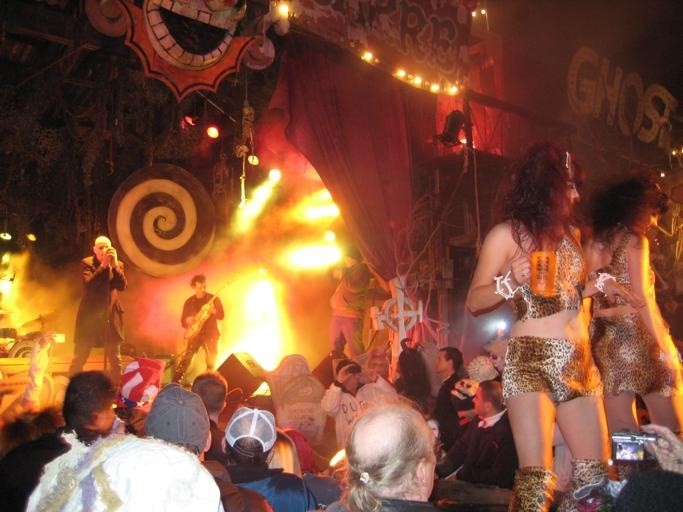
[7,331,52,358]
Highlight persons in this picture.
[66,235,128,379]
[329,246,370,363]
[579,173,683,435]
[1,337,682,512]
[465,144,645,511]
[171,274,225,386]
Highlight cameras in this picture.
[609,430,660,467]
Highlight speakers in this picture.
[216,350,268,400]
[139,356,176,387]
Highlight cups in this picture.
[530,250,558,298]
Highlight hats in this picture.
[345,247,363,262]
[115,358,166,414]
[27,434,226,512]
[336,361,362,375]
[450,379,478,410]
[468,357,499,382]
[483,335,508,354]
[144,383,209,452]
[225,406,277,457]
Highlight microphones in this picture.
[163,361,171,374]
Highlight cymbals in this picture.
[24,313,58,327]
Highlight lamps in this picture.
[433,109,466,150]
